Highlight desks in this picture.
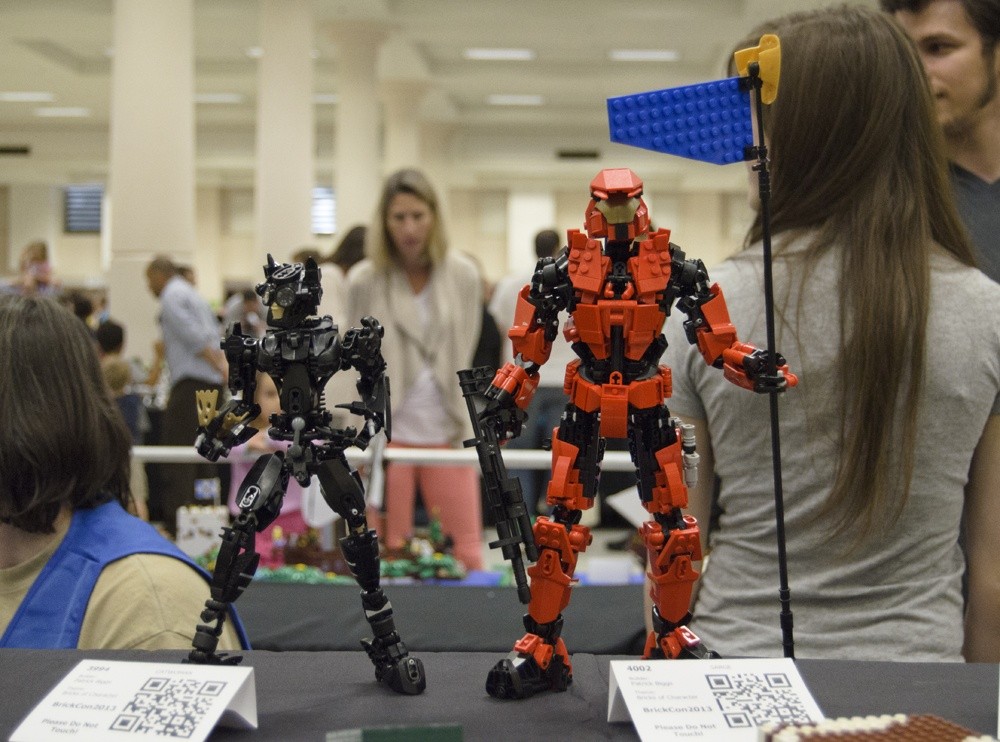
[1,642,1000,741]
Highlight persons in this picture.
[644,4,1000,666]
[852,0,1000,620]
[483,230,580,521]
[471,166,799,698]
[221,366,315,579]
[0,226,238,540]
[337,167,492,584]
[174,250,431,694]
[299,225,376,430]
[196,278,268,415]
[450,266,507,418]
[1,293,255,663]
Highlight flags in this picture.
[605,77,754,164]
